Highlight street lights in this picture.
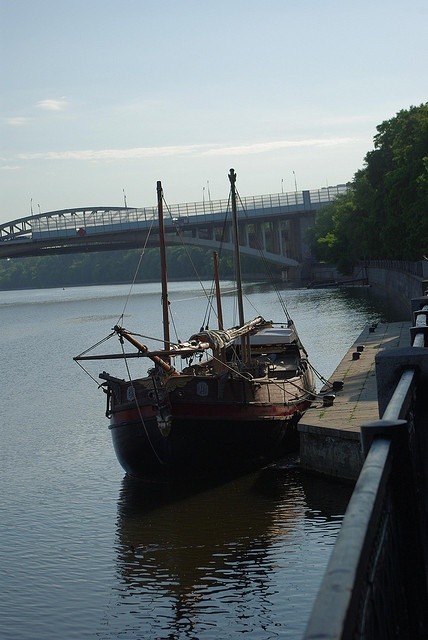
[122,188,128,207]
[291,170,298,191]
[206,180,210,201]
[202,186,205,201]
[36,203,41,214]
[279,178,284,193]
[29,198,33,215]
[123,195,127,207]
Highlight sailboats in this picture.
[72,166,316,488]
